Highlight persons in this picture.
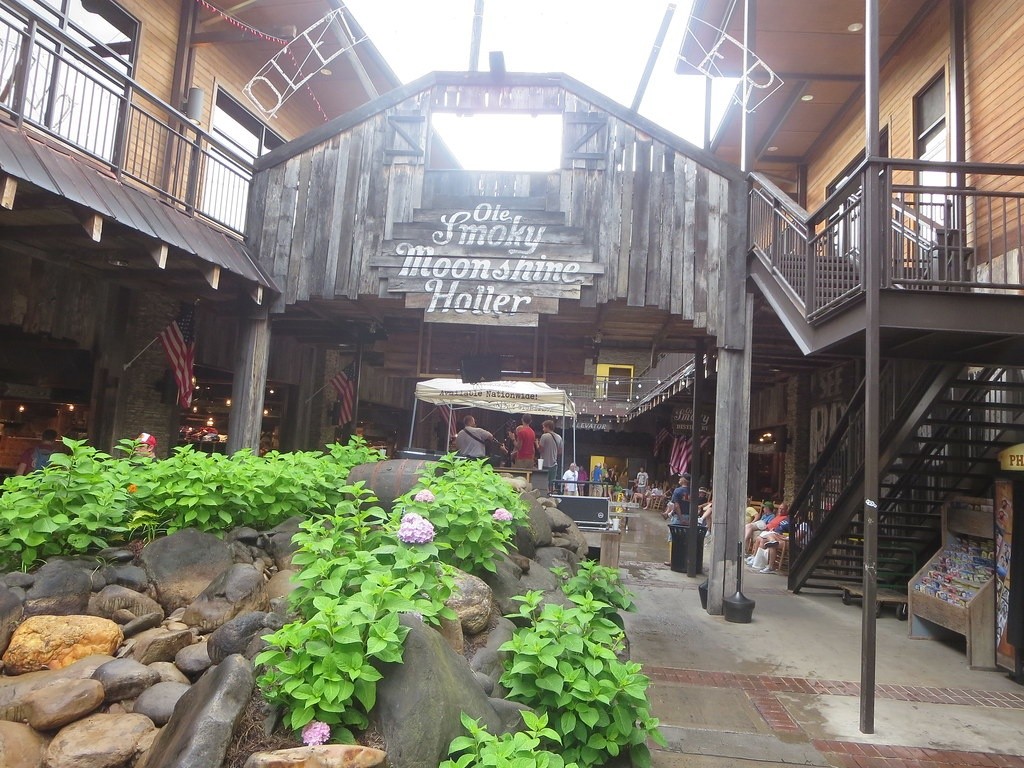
[500,414,535,469]
[536,420,562,494]
[563,463,578,496]
[744,498,812,574]
[135,432,158,464]
[456,415,493,460]
[662,473,713,566]
[577,464,588,496]
[630,466,663,509]
[15,429,58,476]
[591,463,623,502]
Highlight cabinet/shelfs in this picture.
[907,495,996,670]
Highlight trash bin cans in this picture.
[667,525,709,574]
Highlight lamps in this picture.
[320,58,334,76]
[181,87,205,125]
[107,249,129,266]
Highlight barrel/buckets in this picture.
[345,459,447,523]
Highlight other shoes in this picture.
[661,512,667,520]
[668,510,673,518]
[745,556,754,564]
[698,518,704,524]
[699,487,708,493]
[643,506,649,510]
[664,562,671,565]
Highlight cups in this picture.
[380,450,386,456]
[538,459,544,470]
[612,519,619,530]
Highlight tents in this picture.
[408,377,576,495]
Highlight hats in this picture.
[678,473,690,481]
[761,501,773,508]
[130,433,156,448]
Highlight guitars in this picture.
[492,420,516,467]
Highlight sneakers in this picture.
[764,540,779,548]
[760,565,775,574]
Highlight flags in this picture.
[654,423,711,477]
[330,360,356,425]
[158,301,196,408]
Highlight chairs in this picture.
[626,481,789,571]
